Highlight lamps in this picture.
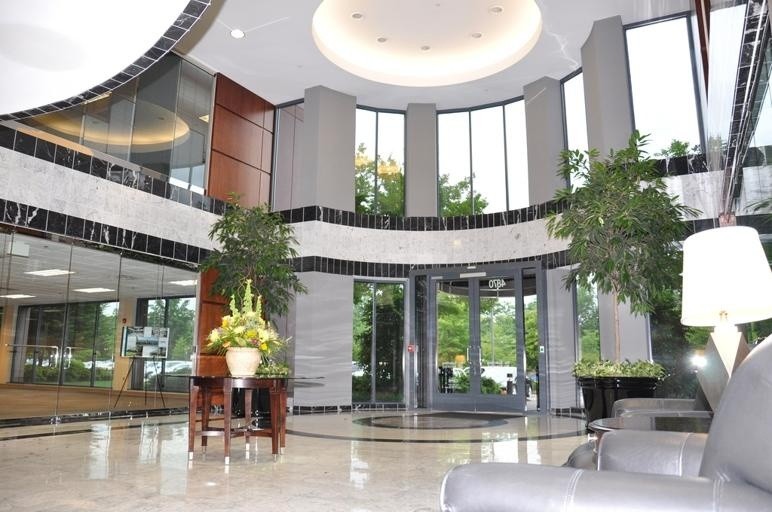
[681,227,772,415]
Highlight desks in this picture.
[188,375,289,466]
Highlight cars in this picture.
[23,352,193,387]
[352,359,533,396]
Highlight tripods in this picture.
[113,358,166,409]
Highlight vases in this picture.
[226,346,262,378]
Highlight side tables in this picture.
[587,417,712,457]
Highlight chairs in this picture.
[612,385,711,417]
[440,334,772,512]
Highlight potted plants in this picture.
[574,359,620,433]
[198,190,310,416]
[597,361,666,416]
[543,129,703,432]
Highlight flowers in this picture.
[204,279,293,362]
